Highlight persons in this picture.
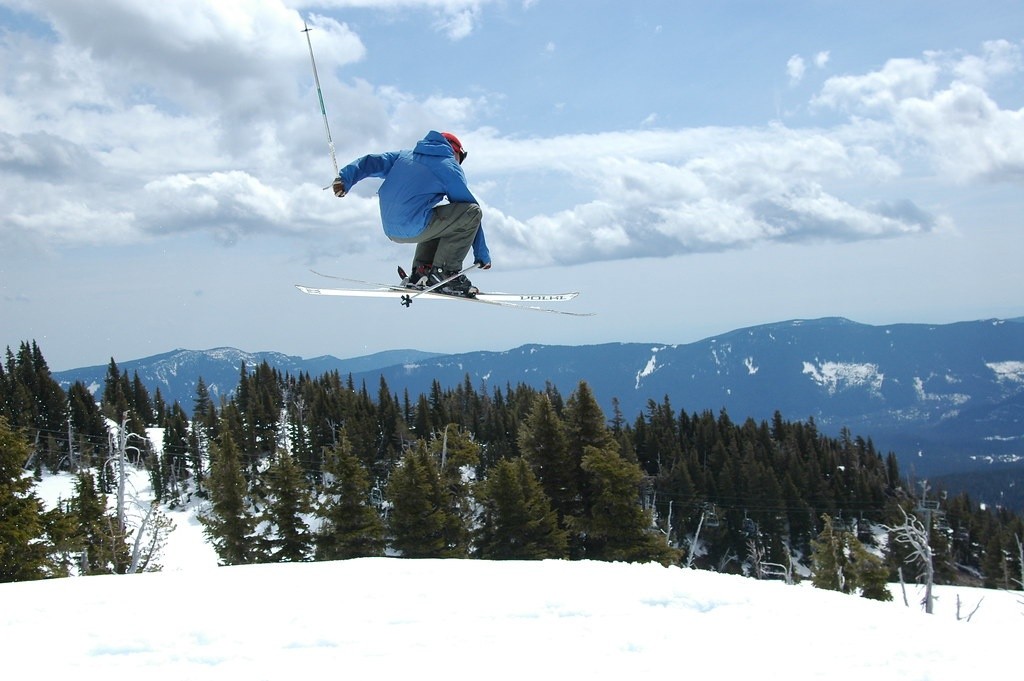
[333,131,492,292]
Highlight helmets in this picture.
[440,132,464,164]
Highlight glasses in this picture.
[459,147,468,163]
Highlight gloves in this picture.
[333,177,346,198]
[474,259,492,269]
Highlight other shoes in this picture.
[425,267,479,298]
[405,266,434,291]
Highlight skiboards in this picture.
[294,267,598,317]
